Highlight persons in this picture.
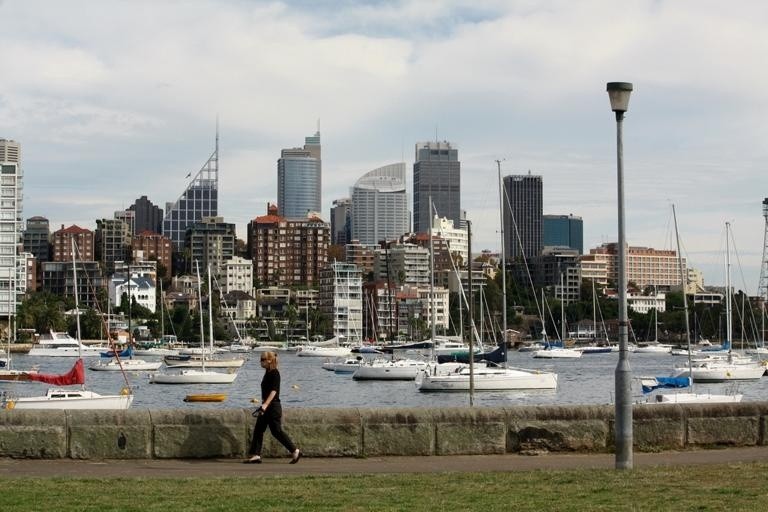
[242,351,302,465]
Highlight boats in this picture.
[583,204,768,405]
[186,394,225,401]
[0,258,279,383]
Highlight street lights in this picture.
[607,82,634,467]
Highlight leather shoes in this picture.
[289,449,303,464]
[244,459,262,464]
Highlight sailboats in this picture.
[1,234,135,410]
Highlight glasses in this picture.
[260,358,266,361]
